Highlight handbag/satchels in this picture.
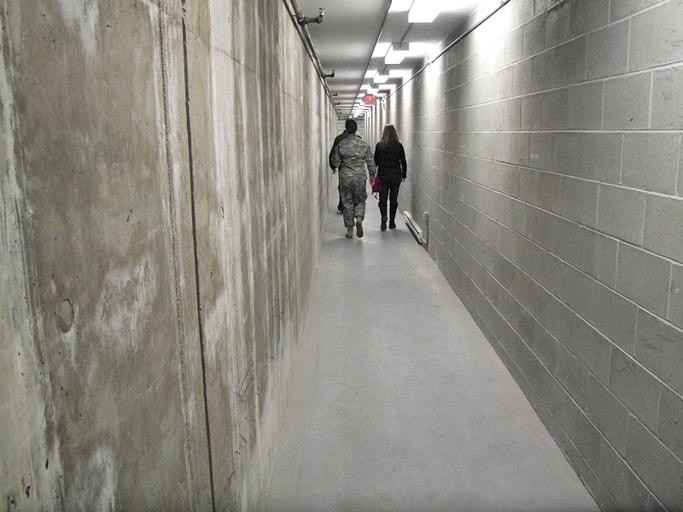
[371,177,382,193]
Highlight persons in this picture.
[330,119,376,240]
[373,125,407,231]
[328,119,362,212]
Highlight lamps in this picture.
[385,42,409,64]
[373,70,389,83]
[367,85,379,94]
[408,0,441,23]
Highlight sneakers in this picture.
[336,209,342,214]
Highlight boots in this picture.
[356,216,363,238]
[345,225,354,239]
[379,203,388,231]
[388,205,398,229]
